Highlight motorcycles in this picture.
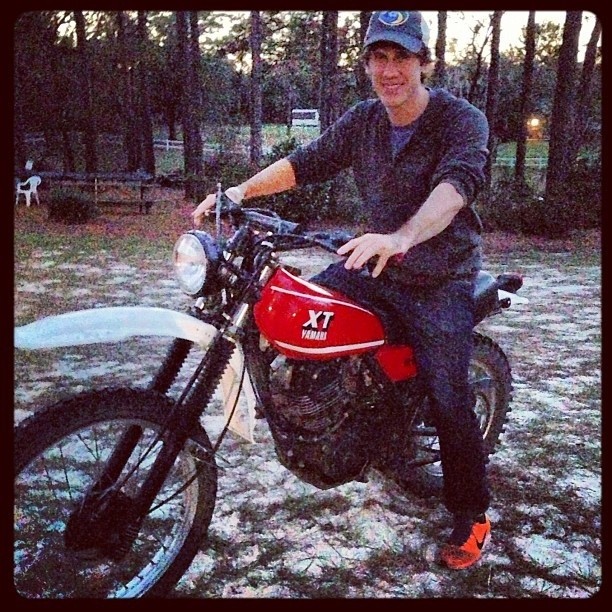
[14,178,525,599]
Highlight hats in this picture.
[363,11,429,53]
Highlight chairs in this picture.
[14,176,42,208]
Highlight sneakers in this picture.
[440,512,491,570]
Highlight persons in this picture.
[190,10,492,571]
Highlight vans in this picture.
[290,108,320,127]
